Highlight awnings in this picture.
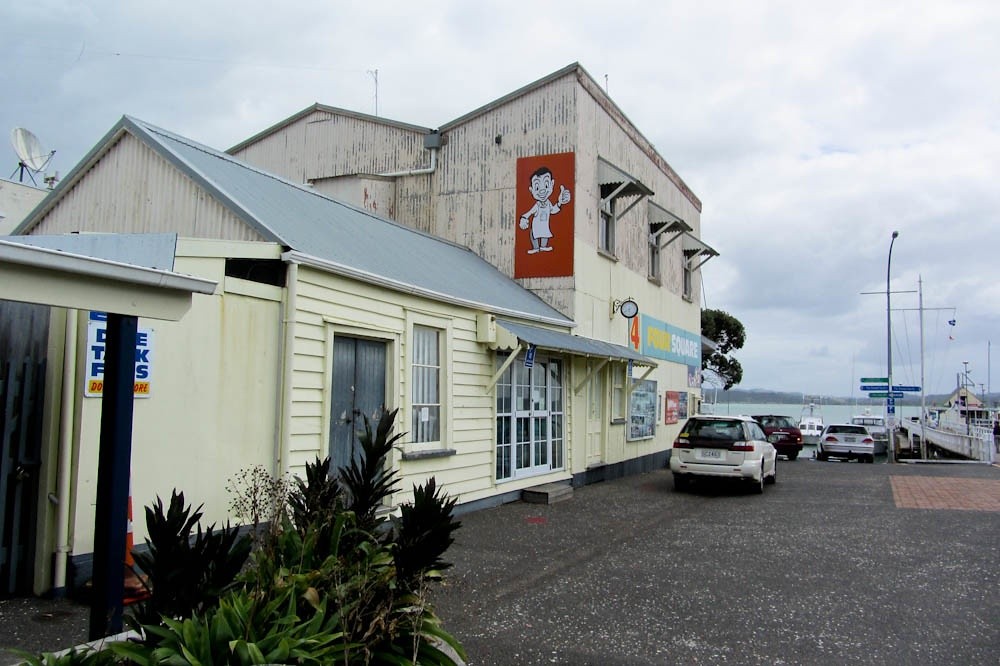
[682,230,720,256]
[483,318,658,369]
[701,334,718,354]
[597,155,655,198]
[648,199,694,233]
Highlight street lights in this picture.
[886,230,899,464]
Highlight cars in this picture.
[750,413,804,460]
[813,424,875,463]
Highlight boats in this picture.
[850,384,889,455]
[901,361,1000,463]
[797,393,825,445]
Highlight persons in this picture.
[993,421,1000,454]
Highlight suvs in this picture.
[669,413,778,494]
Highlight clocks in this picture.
[622,302,637,316]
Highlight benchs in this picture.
[699,426,742,438]
